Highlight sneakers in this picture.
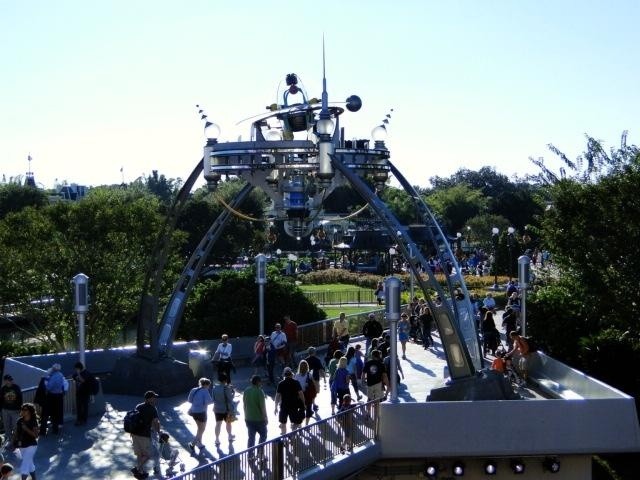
[189,443,195,451]
[229,435,235,440]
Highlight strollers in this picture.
[408,316,422,343]
[495,344,525,390]
[150,428,186,477]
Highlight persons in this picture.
[243,376,269,460]
[525,247,550,268]
[493,331,530,388]
[242,256,255,268]
[456,289,500,356]
[463,250,495,277]
[274,347,327,445]
[503,282,521,348]
[190,334,232,383]
[255,316,299,384]
[35,362,91,435]
[396,257,452,274]
[1,374,40,480]
[398,296,442,359]
[329,312,405,450]
[296,251,390,275]
[131,391,178,478]
[188,372,236,451]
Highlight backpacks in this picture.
[124,408,143,433]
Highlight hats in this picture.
[144,390,159,399]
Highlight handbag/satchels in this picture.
[226,412,238,421]
[288,396,304,424]
[304,373,317,400]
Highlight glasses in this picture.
[221,339,227,341]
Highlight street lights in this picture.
[276,226,339,280]
[525,225,528,236]
[383,278,401,404]
[73,273,89,369]
[467,226,471,252]
[389,230,415,303]
[491,227,499,288]
[507,227,514,284]
[517,255,529,337]
[255,252,266,337]
[457,232,461,246]
